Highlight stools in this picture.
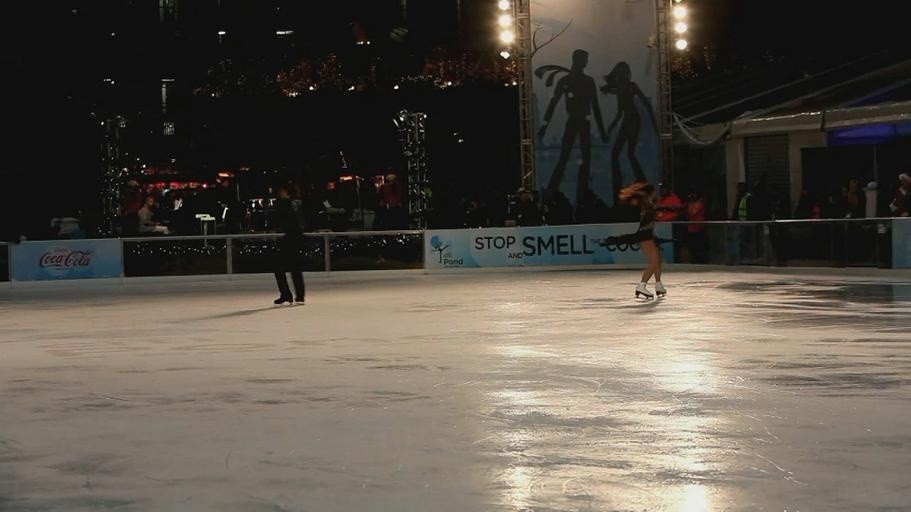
[195,213,216,250]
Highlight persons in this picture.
[261,183,306,304]
[653,173,910,269]
[620,182,666,299]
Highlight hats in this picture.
[898,172,911,182]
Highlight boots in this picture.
[635,282,653,297]
[655,280,666,295]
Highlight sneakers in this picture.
[542,189,603,206]
[275,293,293,304]
[296,296,304,301]
